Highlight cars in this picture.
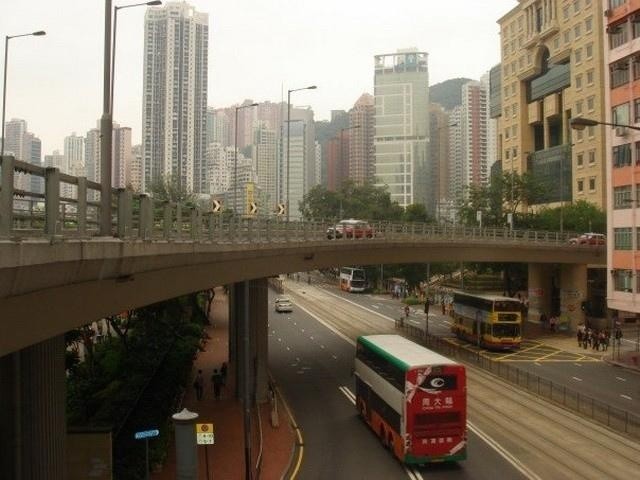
[274,297,294,312]
[568,231,607,247]
[325,219,374,239]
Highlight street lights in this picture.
[110,0,163,129]
[232,103,261,212]
[1,30,47,157]
[284,86,318,219]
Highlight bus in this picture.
[339,266,368,294]
[353,333,468,467]
[449,289,523,352]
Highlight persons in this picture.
[405,306,409,316]
[193,369,204,400]
[211,369,223,400]
[576,325,622,351]
[540,312,556,333]
[220,362,227,379]
